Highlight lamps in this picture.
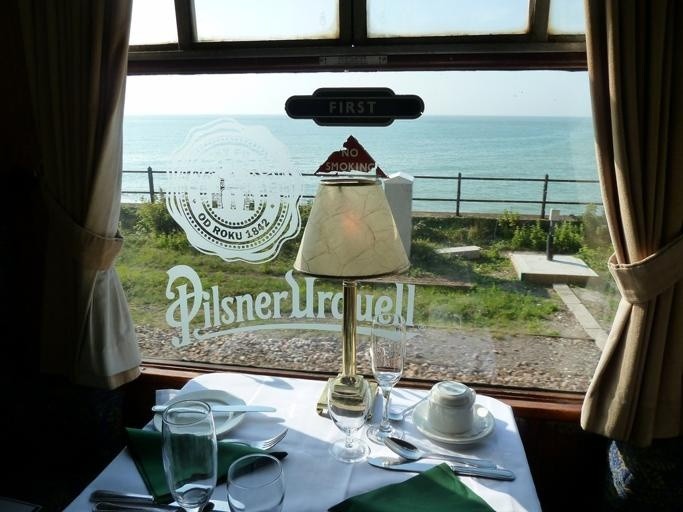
[293,178,412,414]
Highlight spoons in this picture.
[215,426,290,452]
[384,386,432,422]
[383,435,497,470]
[88,489,215,512]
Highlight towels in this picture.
[126,427,289,505]
[328,462,495,512]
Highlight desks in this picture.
[61,372,543,511]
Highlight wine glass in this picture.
[365,312,406,447]
[326,372,371,464]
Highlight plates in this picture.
[153,389,248,437]
[411,398,497,445]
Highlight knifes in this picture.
[150,404,278,412]
[367,456,517,481]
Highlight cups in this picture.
[427,376,477,433]
[159,399,220,511]
[226,453,287,512]
